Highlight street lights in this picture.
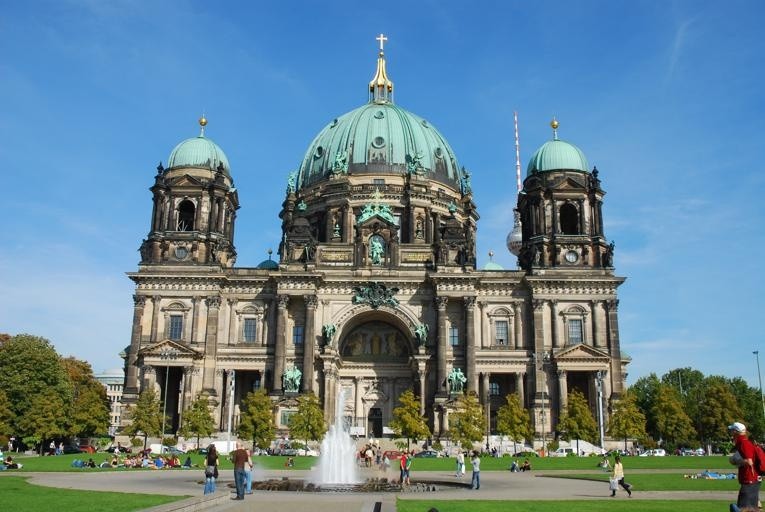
[266,248,274,270]
[250,399,285,453]
[367,437,380,468]
[484,389,492,452]
[156,345,182,455]
[751,349,765,419]
[488,248,496,271]
[529,348,551,457]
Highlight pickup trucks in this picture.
[47,443,94,456]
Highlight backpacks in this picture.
[752,441,765,476]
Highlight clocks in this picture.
[174,245,188,260]
[562,249,581,265]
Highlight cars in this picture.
[384,446,587,459]
[95,443,218,457]
[596,443,707,458]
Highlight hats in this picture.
[727,420,748,434]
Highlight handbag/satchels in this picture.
[203,464,217,476]
[609,475,620,490]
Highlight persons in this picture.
[289,365,302,388]
[468,451,480,489]
[448,368,457,389]
[357,435,498,484]
[0,436,201,471]
[370,237,382,263]
[240,448,253,494]
[203,446,219,496]
[520,458,531,472]
[281,364,293,389]
[609,455,632,497]
[727,421,761,507]
[230,440,248,500]
[413,323,431,345]
[456,367,465,392]
[322,323,337,347]
[511,458,521,472]
[597,455,612,468]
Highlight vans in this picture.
[278,439,318,456]
